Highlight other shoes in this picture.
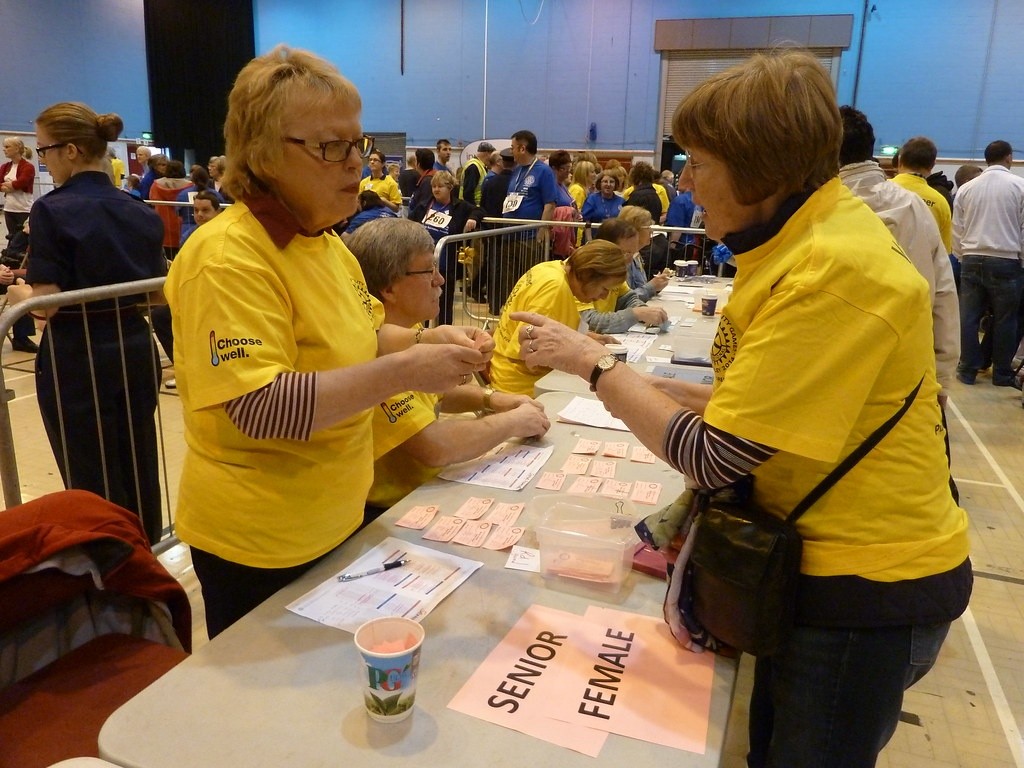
[165,379,176,388]
[13,337,41,353]
[466,286,487,303]
[993,368,1014,386]
[956,368,977,384]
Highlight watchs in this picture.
[482,389,497,416]
[589,353,626,393]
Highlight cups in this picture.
[674,259,688,278]
[687,260,698,276]
[701,293,717,316]
[187,191,199,204]
[355,615,425,723]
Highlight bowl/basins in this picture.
[537,497,636,584]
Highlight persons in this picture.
[0,130,1024,390]
[838,105,963,510]
[489,239,627,401]
[163,43,496,644]
[509,40,975,768]
[340,217,551,528]
[6,102,172,551]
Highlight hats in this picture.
[500,148,514,161]
[477,142,496,152]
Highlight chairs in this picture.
[0,491,192,768]
[474,328,495,389]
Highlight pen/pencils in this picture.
[678,284,703,287]
[337,559,410,582]
[646,323,653,329]
[654,274,673,280]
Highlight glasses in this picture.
[36,143,83,158]
[558,165,571,172]
[284,134,376,163]
[402,265,437,279]
[685,150,714,178]
[623,253,633,259]
[369,158,382,164]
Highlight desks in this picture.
[55,276,742,768]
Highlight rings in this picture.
[525,325,535,339]
[459,375,467,387]
[526,339,535,353]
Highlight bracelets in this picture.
[415,328,430,343]
[27,310,49,321]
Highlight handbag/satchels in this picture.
[679,498,803,660]
